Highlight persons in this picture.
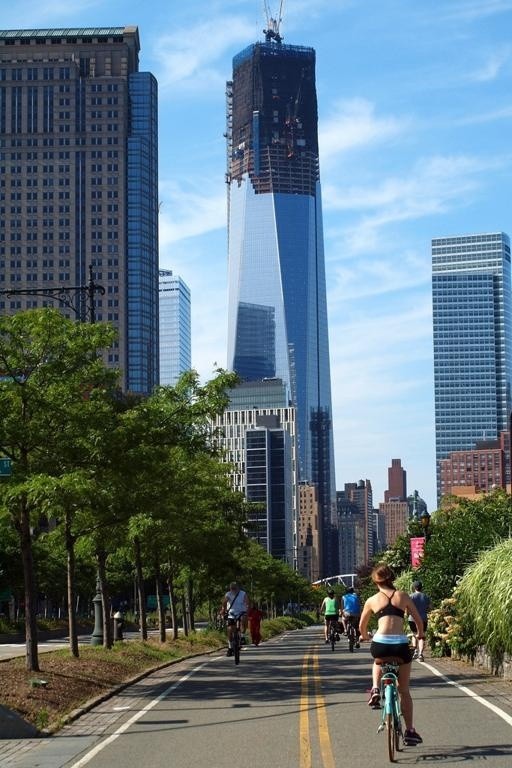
[320,587,361,648]
[219,582,250,656]
[359,563,425,743]
[247,602,264,646]
[406,581,431,662]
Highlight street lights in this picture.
[419,506,432,561]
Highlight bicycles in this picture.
[220,610,247,666]
[361,631,426,762]
[338,609,363,652]
[319,608,341,652]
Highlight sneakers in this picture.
[413,649,424,662]
[240,637,246,645]
[324,631,360,648]
[403,728,423,743]
[226,648,233,657]
[367,687,382,706]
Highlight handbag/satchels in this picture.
[224,613,228,620]
